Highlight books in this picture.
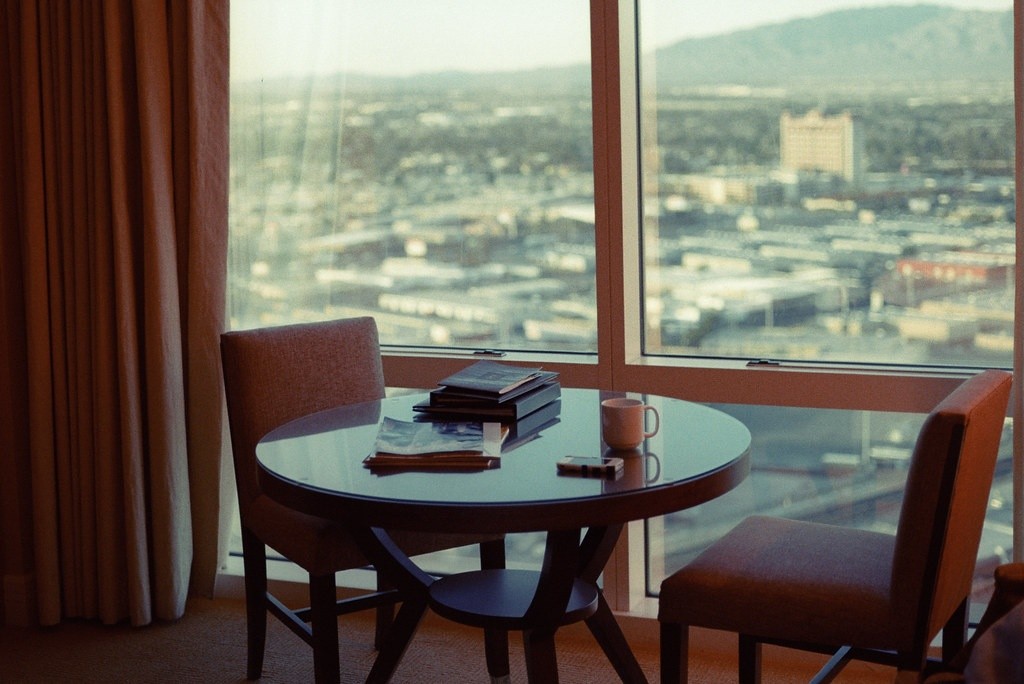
[412,357,562,423]
[499,399,563,453]
[360,414,510,467]
[364,457,502,478]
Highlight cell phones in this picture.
[557,455,623,474]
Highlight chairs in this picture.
[658,368,1012,684]
[217,313,510,684]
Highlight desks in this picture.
[255,388,753,684]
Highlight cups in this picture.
[600,396,661,451]
